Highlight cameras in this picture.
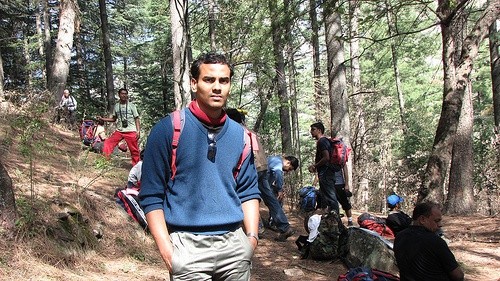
[122,121,128,128]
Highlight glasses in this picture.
[207,130,217,162]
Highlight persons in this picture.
[225,107,466,281]
[55,88,145,190]
[139,51,261,281]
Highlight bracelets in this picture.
[138,131,140,133]
[247,232,258,244]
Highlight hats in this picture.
[387,195,404,206]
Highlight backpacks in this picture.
[296,210,348,260]
[358,212,395,240]
[339,266,400,281]
[325,136,350,171]
[81,119,97,145]
[301,186,317,211]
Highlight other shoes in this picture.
[273,225,295,242]
